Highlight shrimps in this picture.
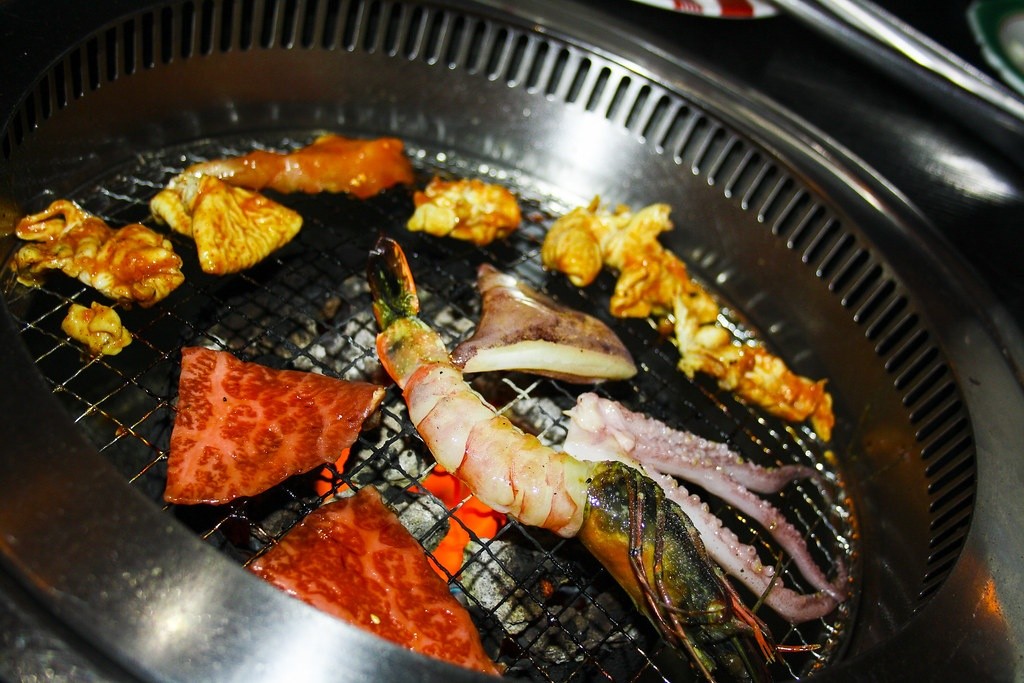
[365,233,823,683]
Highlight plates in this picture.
[966,0,1024,96]
